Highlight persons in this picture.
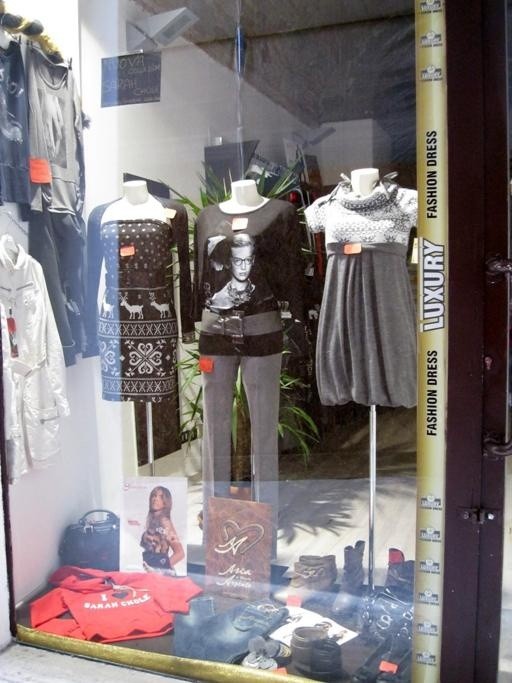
[140,485,185,577]
[202,220,280,315]
[303,166,418,409]
[192,178,306,562]
[79,178,196,403]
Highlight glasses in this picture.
[231,257,254,266]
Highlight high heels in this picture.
[301,554,339,593]
[273,558,333,604]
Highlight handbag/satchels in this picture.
[59,504,120,572]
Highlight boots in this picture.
[332,540,366,616]
